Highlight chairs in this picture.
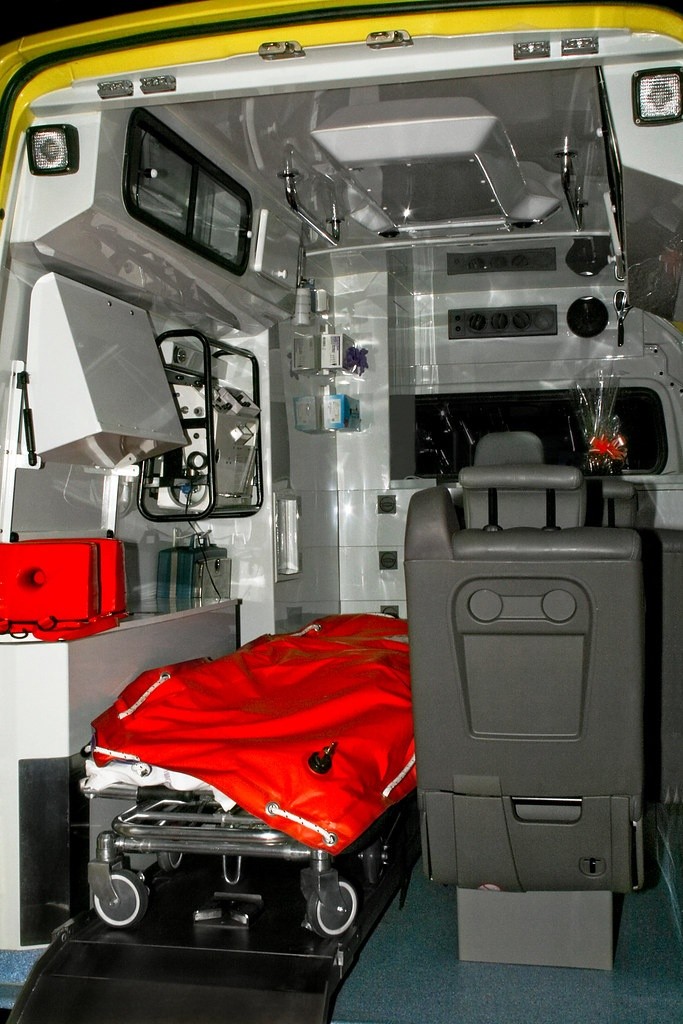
[471,430,542,464]
[402,465,647,894]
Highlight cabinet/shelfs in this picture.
[321,365,364,433]
[290,368,324,434]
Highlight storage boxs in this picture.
[293,396,321,432]
[323,394,361,431]
[322,334,360,374]
[291,336,320,372]
[159,543,228,602]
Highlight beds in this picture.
[80,611,418,939]
